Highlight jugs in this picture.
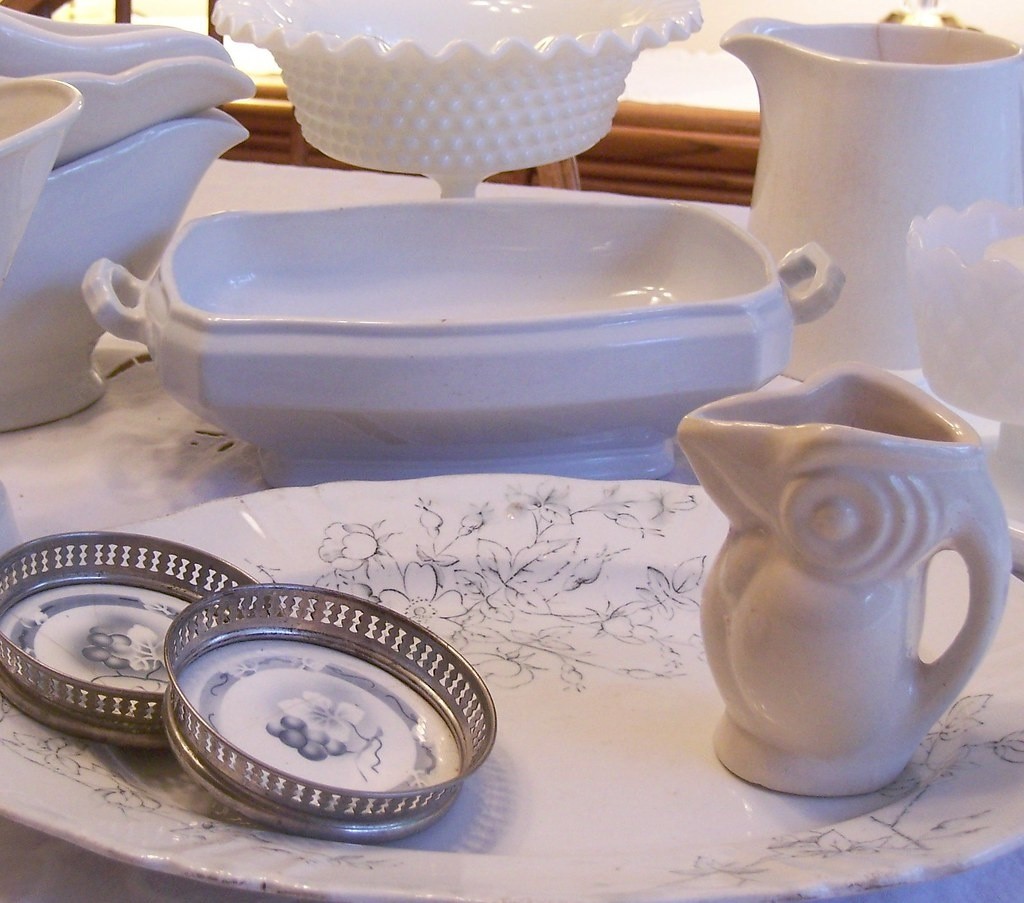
[0,7,256,432]
[675,358,1012,799]
[719,17,1024,383]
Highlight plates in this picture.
[84,194,845,490]
[0,470,1024,903]
